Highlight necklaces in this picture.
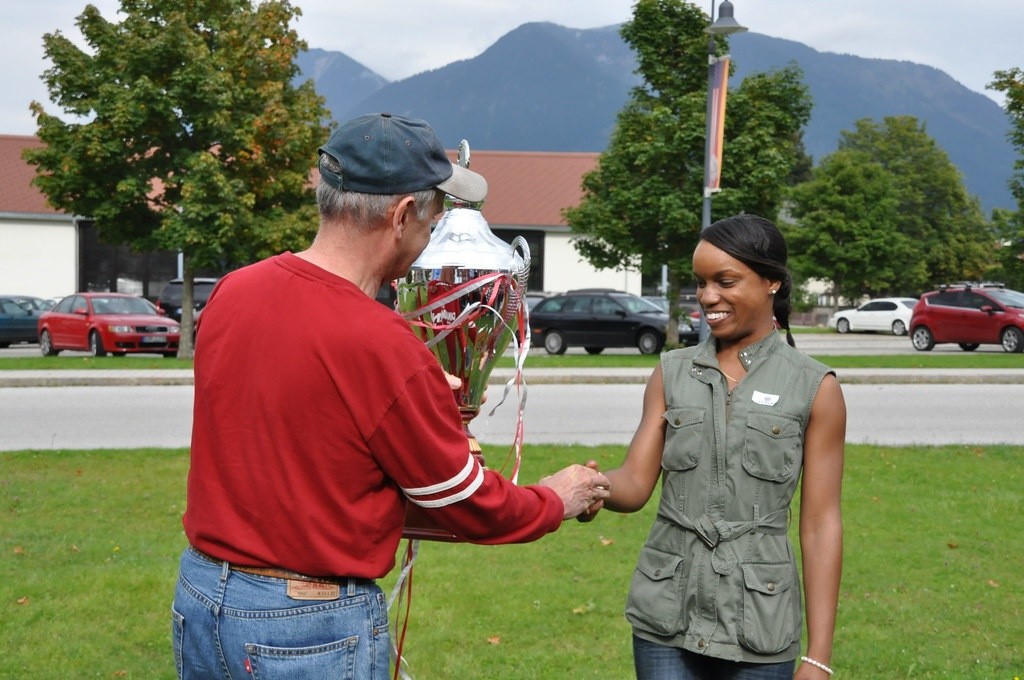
[722,371,739,383]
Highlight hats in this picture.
[319,113,488,202]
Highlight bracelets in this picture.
[801,656,834,675]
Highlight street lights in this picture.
[701,1,748,231]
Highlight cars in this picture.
[509,291,583,347]
[910,282,1024,352]
[0,295,56,349]
[829,296,919,336]
[37,293,181,358]
[157,279,220,330]
[528,289,703,357]
[644,294,702,319]
[690,311,781,331]
[377,283,400,309]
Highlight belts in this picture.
[191,546,376,586]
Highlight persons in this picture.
[576,214,846,680]
[171,112,611,680]
